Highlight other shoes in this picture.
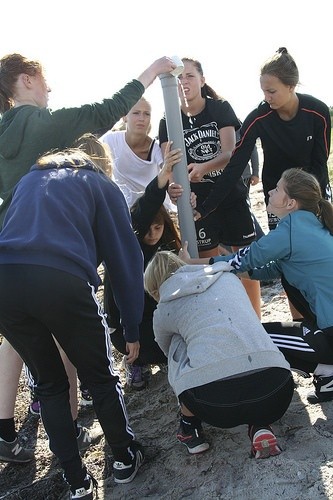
[81,388,92,404]
[29,401,42,421]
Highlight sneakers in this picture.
[112,440,145,483]
[127,363,151,390]
[74,425,101,450]
[176,419,210,453]
[307,374,333,403]
[69,469,98,500]
[0,437,34,462]
[248,424,282,459]
[158,362,168,373]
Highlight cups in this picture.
[169,55,183,76]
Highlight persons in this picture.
[0,133,146,500]
[0,47,333,462]
